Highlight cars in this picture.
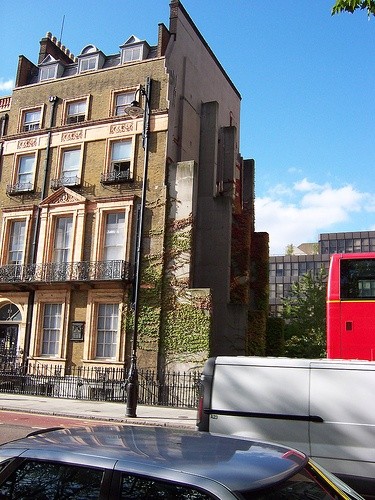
[0,423,360,500]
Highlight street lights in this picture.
[123,76,153,417]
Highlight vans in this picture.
[199,354,375,500]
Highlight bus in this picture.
[325,252,374,362]
